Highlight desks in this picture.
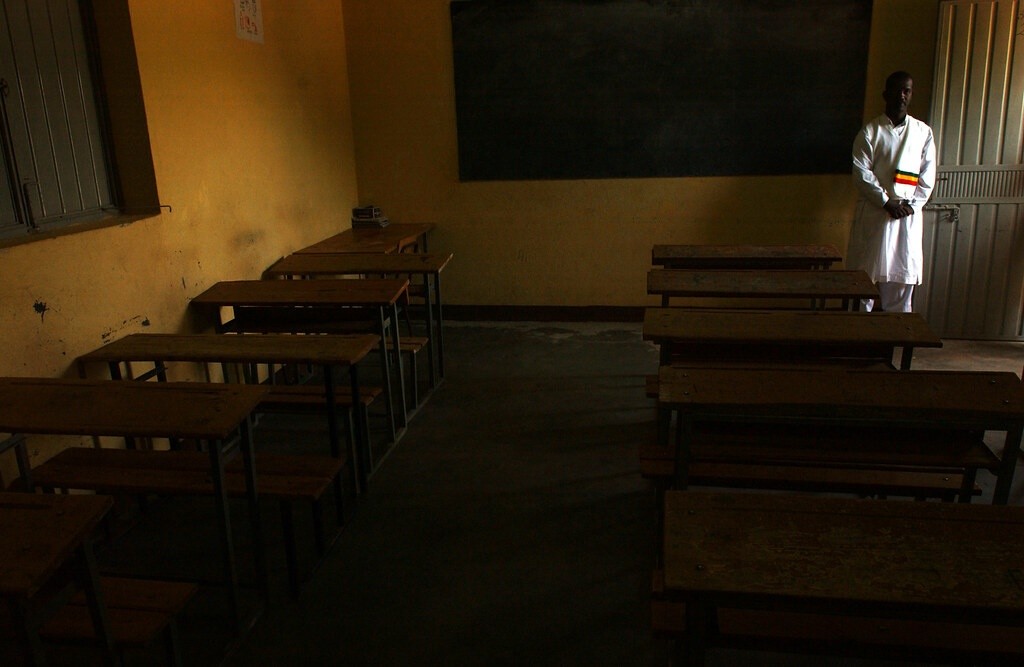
[650,244,841,272]
[78,334,382,525]
[658,366,1024,504]
[647,270,880,307]
[0,378,274,633]
[190,278,412,444]
[262,250,453,389]
[293,223,437,320]
[0,491,123,667]
[643,306,942,369]
[664,487,1024,667]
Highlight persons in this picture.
[846,71,937,312]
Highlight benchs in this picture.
[371,336,429,409]
[638,444,982,522]
[409,285,433,303]
[652,568,1024,667]
[645,370,928,399]
[37,577,200,667]
[9,447,346,594]
[262,385,384,494]
